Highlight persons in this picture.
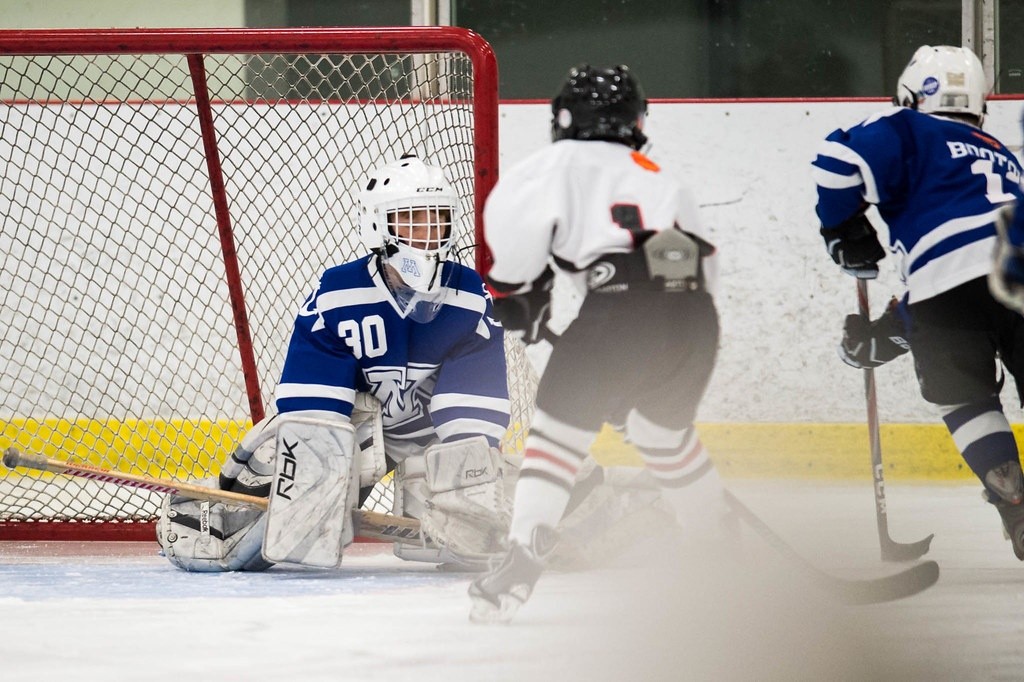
[468,64,723,624]
[155,155,611,573]
[810,45,1024,560]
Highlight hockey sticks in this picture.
[4,445,646,557]
[538,325,945,608]
[854,276,935,563]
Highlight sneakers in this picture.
[469,525,561,624]
[982,459,1024,561]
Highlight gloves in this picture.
[838,295,912,370]
[485,264,556,345]
[821,214,886,280]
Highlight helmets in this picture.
[550,62,648,152]
[360,157,458,294]
[892,45,985,122]
